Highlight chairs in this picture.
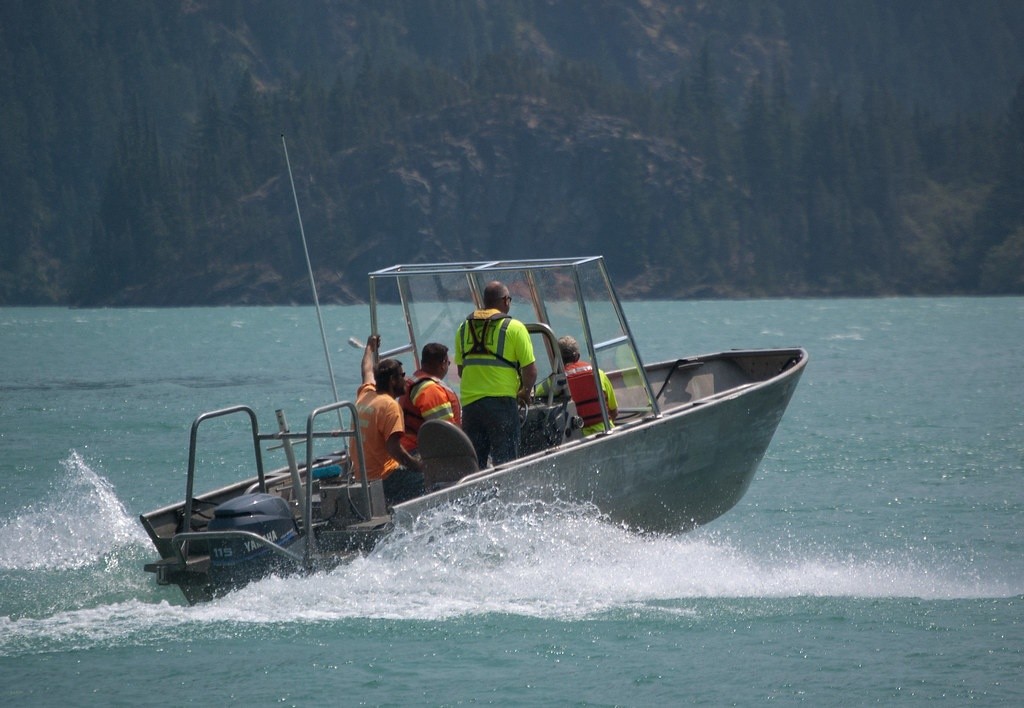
[417,419,480,491]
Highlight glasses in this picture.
[393,372,405,377]
[503,297,511,302]
[442,360,451,365]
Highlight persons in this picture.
[397,342,460,457]
[537,335,618,437]
[453,281,538,471]
[348,335,426,505]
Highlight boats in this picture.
[139,134,809,606]
